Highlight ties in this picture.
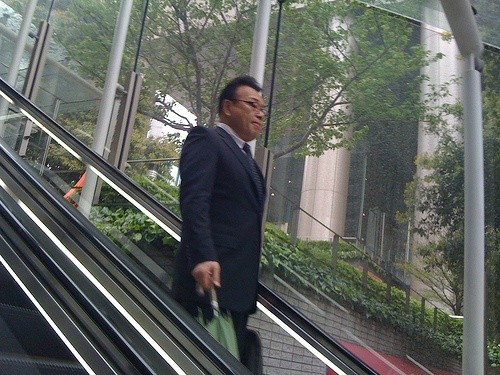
[242,143,262,202]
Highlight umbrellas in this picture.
[195,271,239,361]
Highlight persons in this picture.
[171,76,267,364]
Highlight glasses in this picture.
[234,99,267,117]
